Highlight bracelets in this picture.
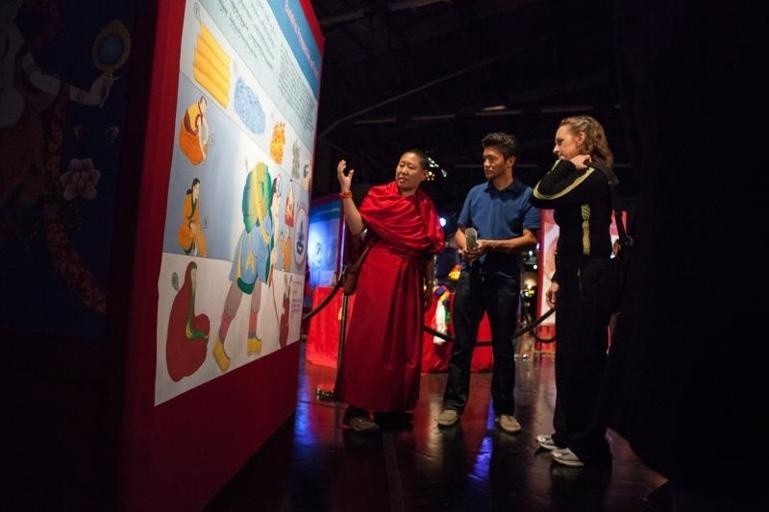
[340,191,352,198]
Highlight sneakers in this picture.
[535,434,584,467]
[495,412,521,434]
[343,404,415,432]
[437,408,460,427]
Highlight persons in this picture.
[336,149,445,433]
[613,241,621,256]
[529,116,616,467]
[437,134,540,433]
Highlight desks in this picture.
[307,286,494,373]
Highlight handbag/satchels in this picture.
[573,259,626,317]
[344,264,361,295]
